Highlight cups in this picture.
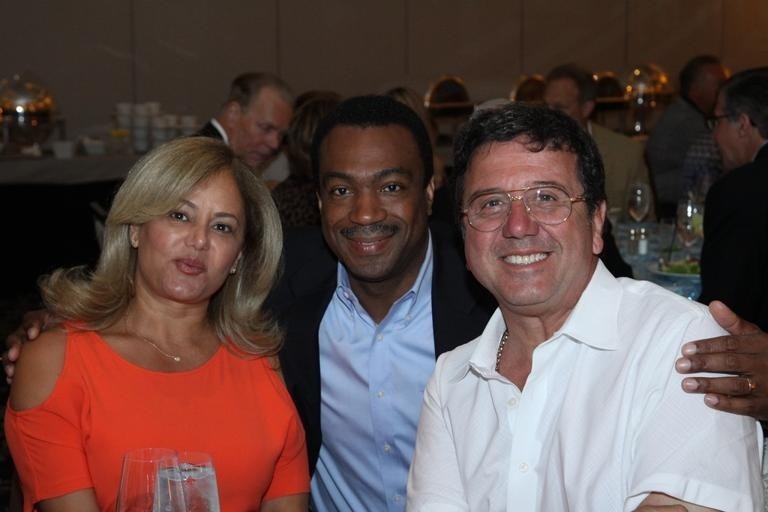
[116,447,187,512]
[154,450,222,511]
[115,102,203,154]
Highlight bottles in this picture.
[627,226,648,253]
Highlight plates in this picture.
[646,262,700,281]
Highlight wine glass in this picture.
[627,182,650,241]
[676,200,701,262]
[606,208,623,236]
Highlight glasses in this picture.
[459,185,591,233]
[706,113,735,132]
